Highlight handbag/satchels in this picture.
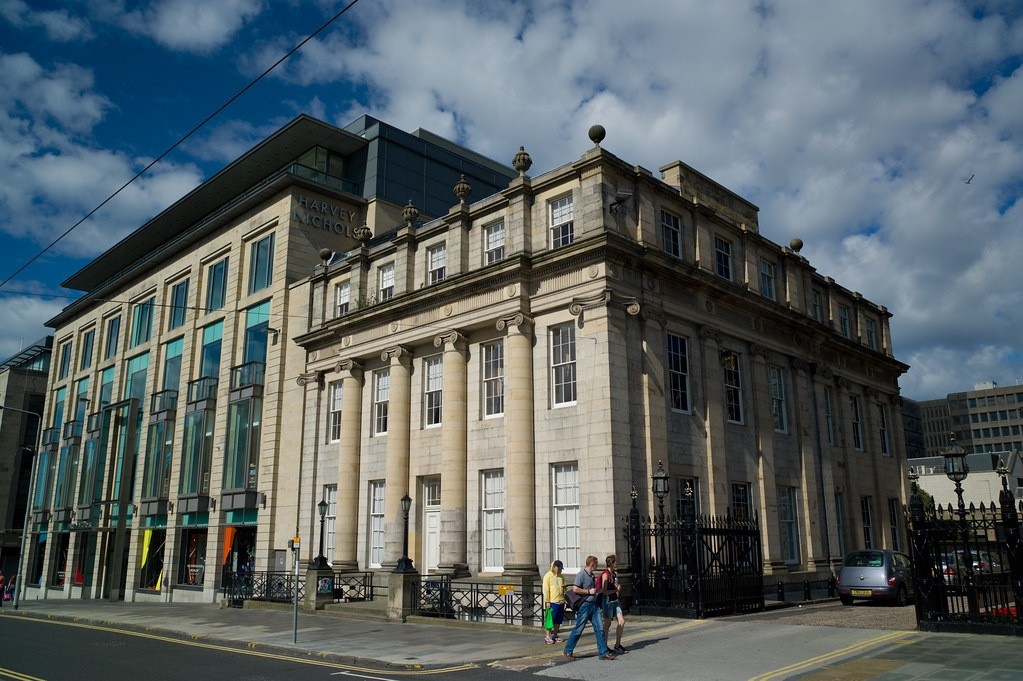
[564,588,584,610]
[543,607,554,630]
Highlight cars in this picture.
[930,552,967,594]
[835,549,914,607]
[953,550,1008,582]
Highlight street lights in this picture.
[939,429,984,623]
[389,491,421,574]
[0,404,42,610]
[650,458,675,607]
[305,496,332,570]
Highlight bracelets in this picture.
[588,589,590,595]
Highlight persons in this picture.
[542,560,568,645]
[0,571,4,607]
[8,575,16,602]
[597,555,629,654]
[563,556,617,661]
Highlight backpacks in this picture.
[595,570,610,593]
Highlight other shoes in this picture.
[545,636,628,660]
